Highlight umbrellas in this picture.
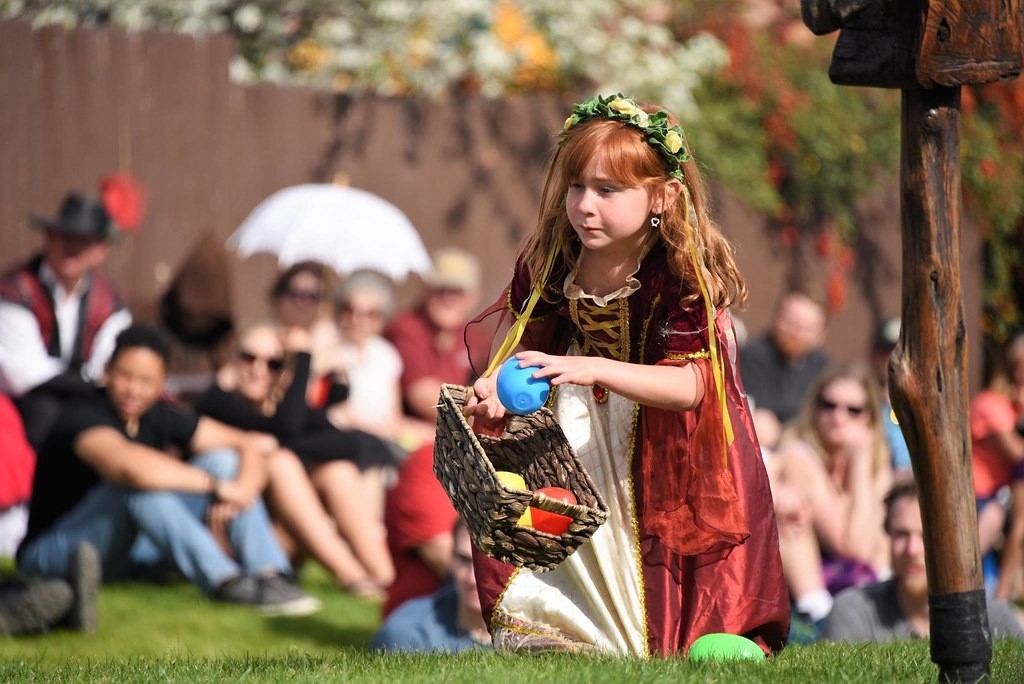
[222,182,432,283]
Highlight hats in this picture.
[424,251,483,290]
[30,191,129,247]
[868,316,904,355]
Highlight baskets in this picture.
[432,383,610,573]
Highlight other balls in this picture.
[688,631,767,669]
[528,486,577,537]
[495,351,549,416]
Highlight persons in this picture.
[464,99,792,659]
[725,294,1024,643]
[0,188,498,654]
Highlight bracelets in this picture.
[210,475,219,494]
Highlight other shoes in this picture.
[47,541,98,633]
[0,578,70,635]
[212,573,321,617]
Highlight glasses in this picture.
[278,287,324,306]
[818,397,874,418]
